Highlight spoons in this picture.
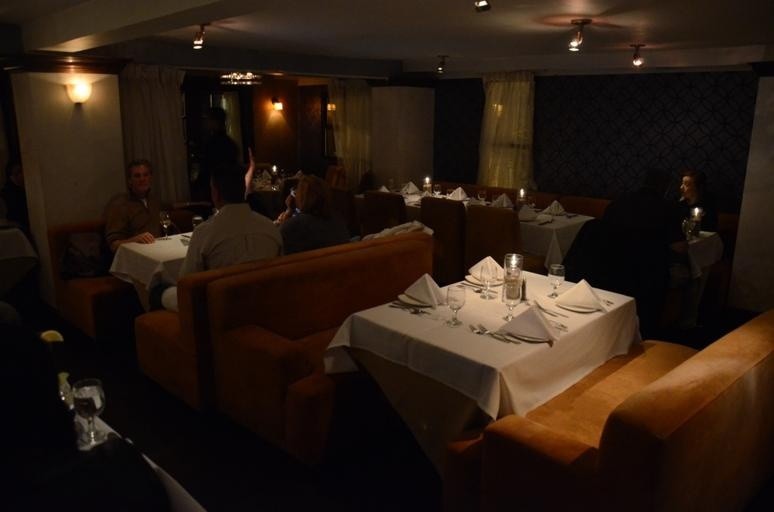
[455,283,482,293]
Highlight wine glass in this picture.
[479,262,499,301]
[157,210,171,240]
[445,285,467,329]
[432,183,536,212]
[546,263,564,298]
[70,376,108,443]
[500,278,523,321]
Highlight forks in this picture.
[389,301,431,316]
[469,323,520,344]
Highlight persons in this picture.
[195,106,240,203]
[2,317,177,511]
[558,163,722,338]
[1,162,30,228]
[101,160,168,315]
[271,175,351,257]
[159,163,282,314]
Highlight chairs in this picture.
[320,167,718,283]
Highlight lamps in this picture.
[569,19,592,51]
[433,55,448,76]
[272,95,282,110]
[193,24,205,50]
[67,79,91,110]
[630,44,646,66]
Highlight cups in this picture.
[191,217,203,230]
[502,253,524,277]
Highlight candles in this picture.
[423,177,432,195]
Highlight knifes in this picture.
[525,299,570,318]
[461,282,497,294]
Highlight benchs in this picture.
[135,230,423,416]
[483,308,773,512]
[46,201,209,339]
[204,234,434,468]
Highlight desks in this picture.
[323,268,641,420]
[108,231,193,290]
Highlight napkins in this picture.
[490,308,554,346]
[559,279,607,313]
[468,255,505,281]
[398,273,448,308]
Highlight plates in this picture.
[464,274,501,286]
[556,303,596,313]
[397,293,432,306]
[509,330,544,343]
